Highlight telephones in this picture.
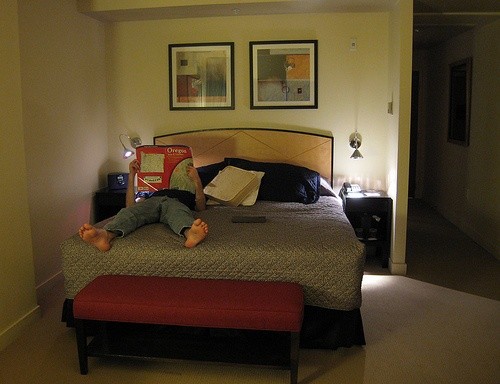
[343,182,361,193]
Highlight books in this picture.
[136,144,195,194]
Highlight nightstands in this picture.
[341,187,392,267]
[96,186,127,225]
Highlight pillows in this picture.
[320,177,336,197]
[223,158,320,204]
[206,170,266,206]
[196,161,225,188]
[203,165,259,208]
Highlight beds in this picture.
[59,128,367,351]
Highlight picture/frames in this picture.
[249,40,318,110]
[446,57,472,147]
[168,42,235,110]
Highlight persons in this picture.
[77,159,208,252]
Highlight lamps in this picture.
[118,135,142,159]
[350,136,364,159]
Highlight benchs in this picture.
[72,274,303,384]
